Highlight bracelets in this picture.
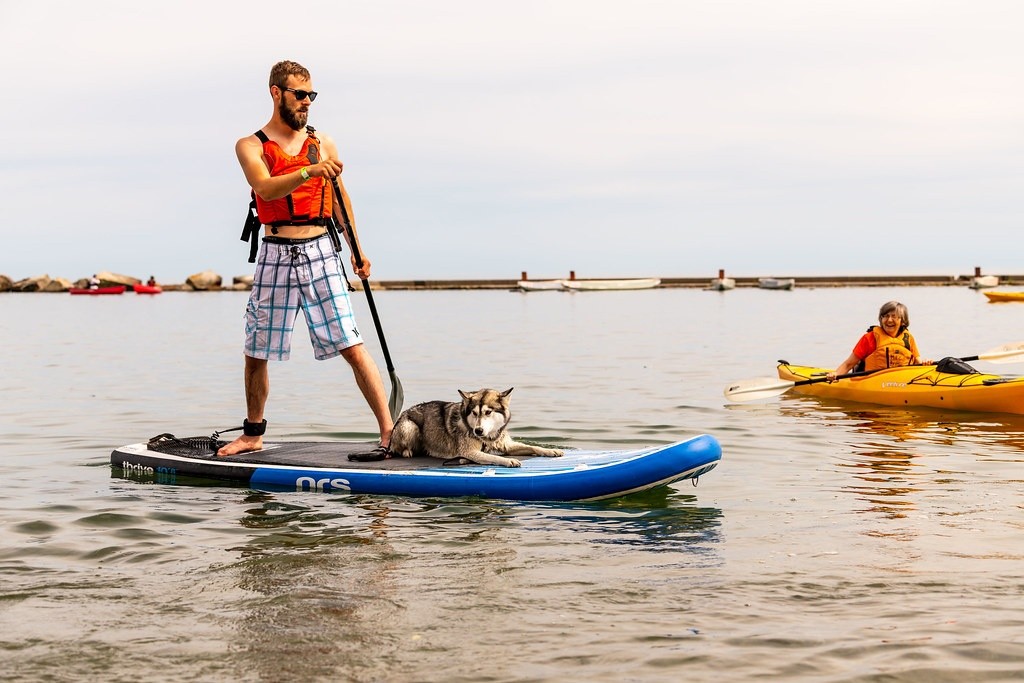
[301,166,313,181]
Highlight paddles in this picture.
[329,168,404,422]
[723,342,1024,404]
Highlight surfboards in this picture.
[110,433,724,503]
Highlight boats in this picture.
[757,277,797,291]
[134,283,163,294]
[711,275,742,290]
[776,358,1024,415]
[69,285,125,295]
[517,278,662,291]
[969,275,1000,290]
[982,290,1024,302]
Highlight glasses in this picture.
[276,85,318,101]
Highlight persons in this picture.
[148,276,156,286]
[218,59,396,458]
[90,274,100,289]
[827,300,933,382]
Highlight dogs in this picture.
[388,387,565,467]
